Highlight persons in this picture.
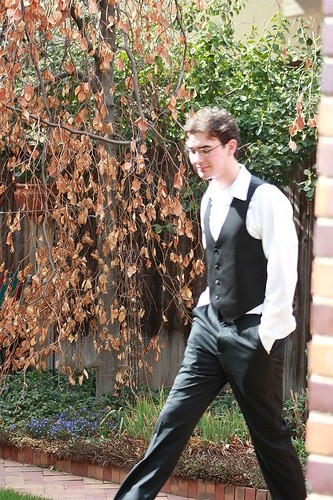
[112,106,310,500]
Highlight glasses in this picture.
[187,141,228,157]
